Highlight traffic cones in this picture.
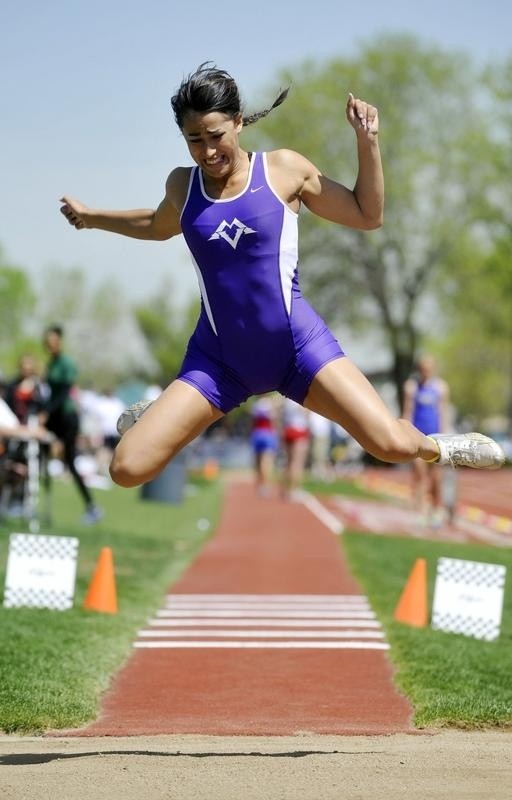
[395,558,428,628]
[84,544,117,614]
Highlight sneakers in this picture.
[117,401,155,438]
[79,510,103,529]
[426,432,505,472]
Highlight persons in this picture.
[1,327,363,534]
[402,355,449,525]
[56,59,508,491]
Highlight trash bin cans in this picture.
[142,451,186,502]
